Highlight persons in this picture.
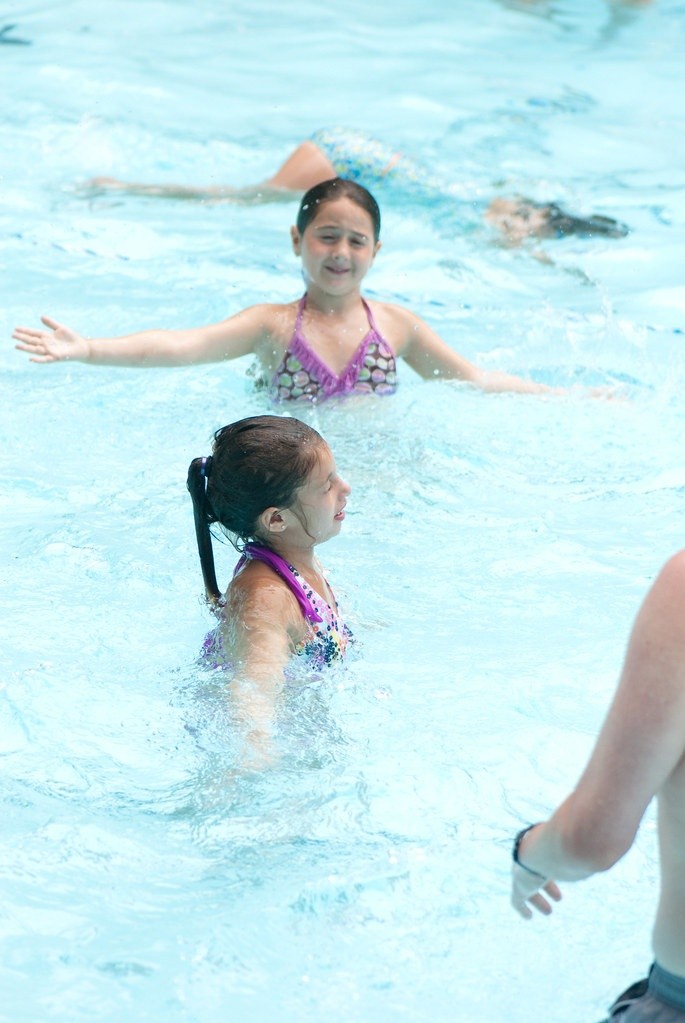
[510,549,685,1023]
[13,175,473,401]
[187,416,358,659]
[270,126,427,190]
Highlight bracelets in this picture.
[512,823,546,878]
